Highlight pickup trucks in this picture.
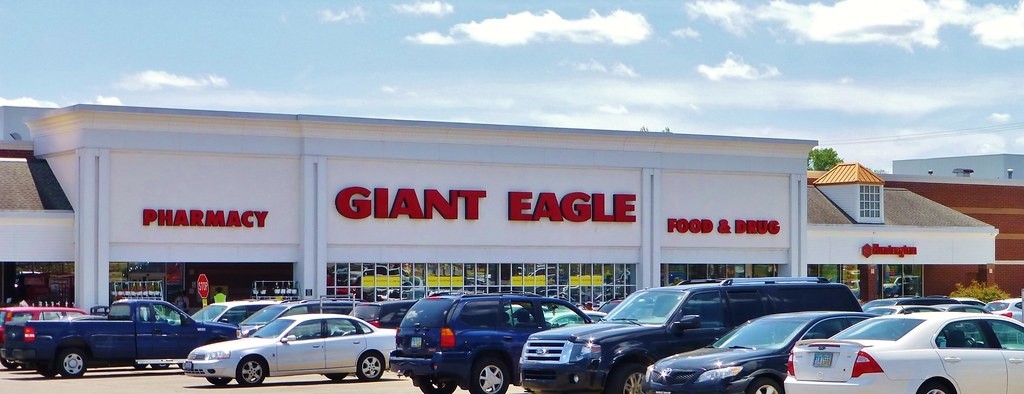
[0,298,244,379]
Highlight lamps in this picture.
[12,132,23,141]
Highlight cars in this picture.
[783,311,1024,394]
[641,311,882,394]
[182,314,397,387]
[502,297,628,327]
[844,279,861,300]
[323,265,613,301]
[861,293,1024,346]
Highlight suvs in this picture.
[344,300,418,329]
[518,276,868,394]
[883,274,920,298]
[237,298,361,338]
[389,289,597,394]
[64,299,289,329]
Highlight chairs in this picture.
[513,308,538,329]
[948,330,966,347]
[652,295,677,317]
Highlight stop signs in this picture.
[197,274,210,298]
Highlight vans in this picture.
[0,306,91,369]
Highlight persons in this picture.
[584,302,594,310]
[211,287,227,302]
[174,291,189,309]
